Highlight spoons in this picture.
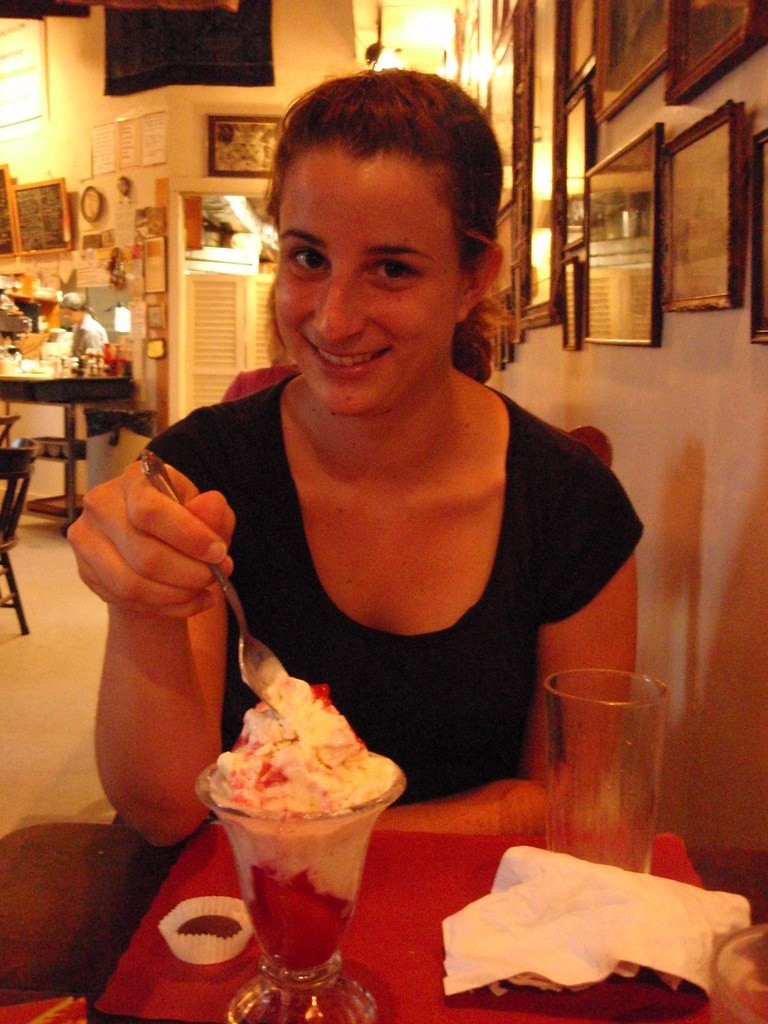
[141,448,289,712]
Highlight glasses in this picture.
[61,311,76,319]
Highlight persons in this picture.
[64,68,646,992]
[57,292,109,359]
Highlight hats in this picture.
[60,292,85,310]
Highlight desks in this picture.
[1,780,768,1024]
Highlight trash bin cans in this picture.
[82,407,158,492]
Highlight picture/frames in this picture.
[453,0,768,368]
[207,115,280,180]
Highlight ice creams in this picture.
[204,673,398,916]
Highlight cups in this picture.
[711,923,767,1023]
[543,669,669,875]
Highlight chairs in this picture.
[0,430,41,635]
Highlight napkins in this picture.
[440,844,751,998]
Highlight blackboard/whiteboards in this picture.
[0,164,17,258]
[11,178,72,256]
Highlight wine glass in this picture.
[195,752,408,1024]
